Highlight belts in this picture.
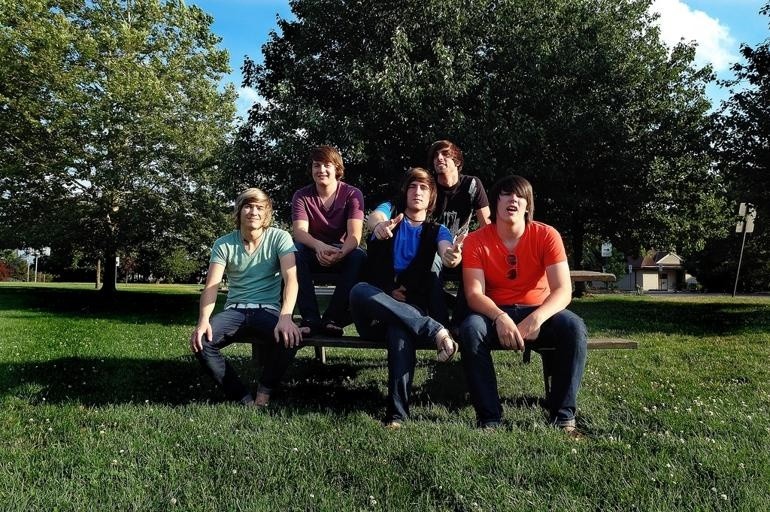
[225,303,280,311]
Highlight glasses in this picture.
[505,254,517,281]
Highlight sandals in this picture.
[298,318,320,337]
[321,318,344,337]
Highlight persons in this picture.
[348,169,464,430]
[461,176,587,438]
[291,144,366,338]
[366,141,492,327]
[191,188,304,407]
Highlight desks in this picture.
[298,269,618,363]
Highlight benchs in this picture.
[219,317,639,405]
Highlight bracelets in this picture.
[373,221,384,231]
[495,313,506,322]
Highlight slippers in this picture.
[433,328,458,364]
